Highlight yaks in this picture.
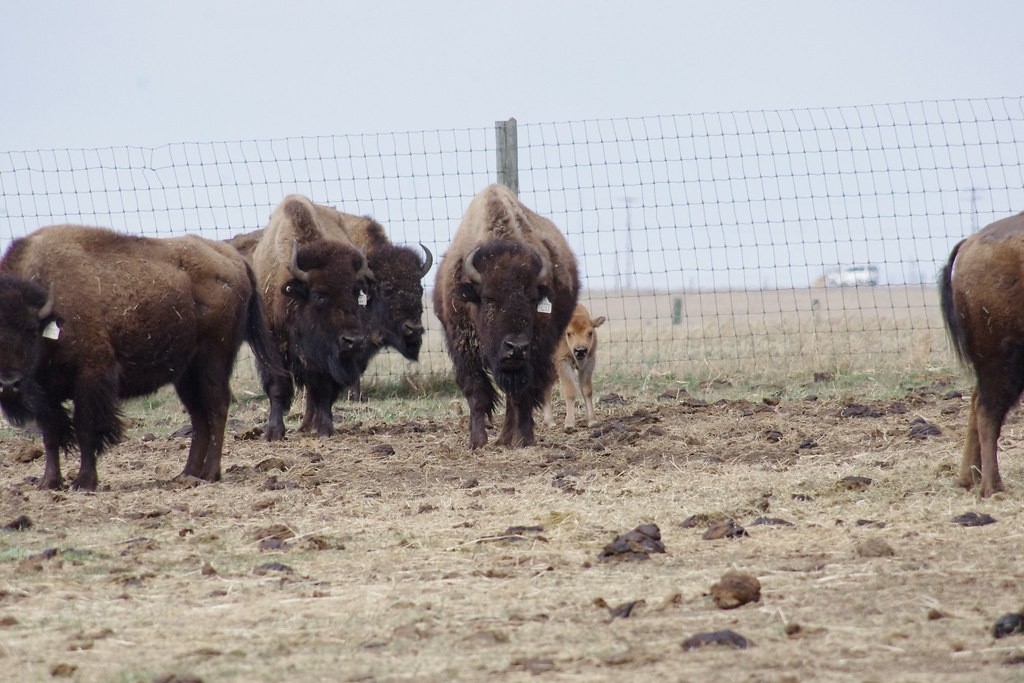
[0,184,605,492]
[938,210,1024,499]
